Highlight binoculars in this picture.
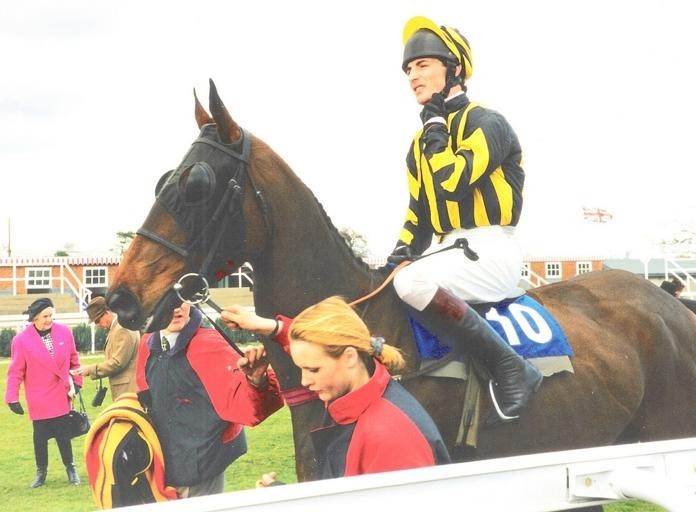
[92,386,107,407]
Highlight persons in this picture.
[134,302,284,497]
[4,298,83,488]
[74,296,141,402]
[377,14,543,426]
[220,296,451,482]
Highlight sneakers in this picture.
[30,469,46,487]
[67,464,80,484]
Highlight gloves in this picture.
[420,92,448,127]
[9,401,24,415]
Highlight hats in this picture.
[28,299,52,320]
[86,297,107,321]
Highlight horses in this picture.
[105,77,696,483]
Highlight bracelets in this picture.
[269,318,279,336]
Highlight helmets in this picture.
[401,16,474,78]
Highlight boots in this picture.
[416,284,544,420]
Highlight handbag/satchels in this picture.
[68,390,91,439]
